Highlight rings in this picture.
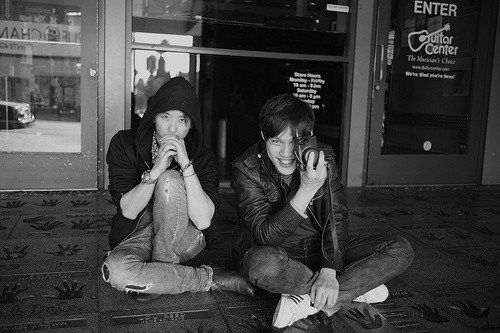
[174,136,180,142]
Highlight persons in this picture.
[101,76,257,298]
[230,95,415,330]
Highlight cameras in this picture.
[294,135,318,171]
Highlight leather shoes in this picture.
[209,258,255,298]
[135,293,153,303]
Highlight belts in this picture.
[280,237,319,251]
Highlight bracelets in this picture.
[180,160,196,177]
[141,171,157,184]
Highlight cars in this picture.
[0,101,35,129]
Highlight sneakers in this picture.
[271,293,319,329]
[352,284,390,304]
